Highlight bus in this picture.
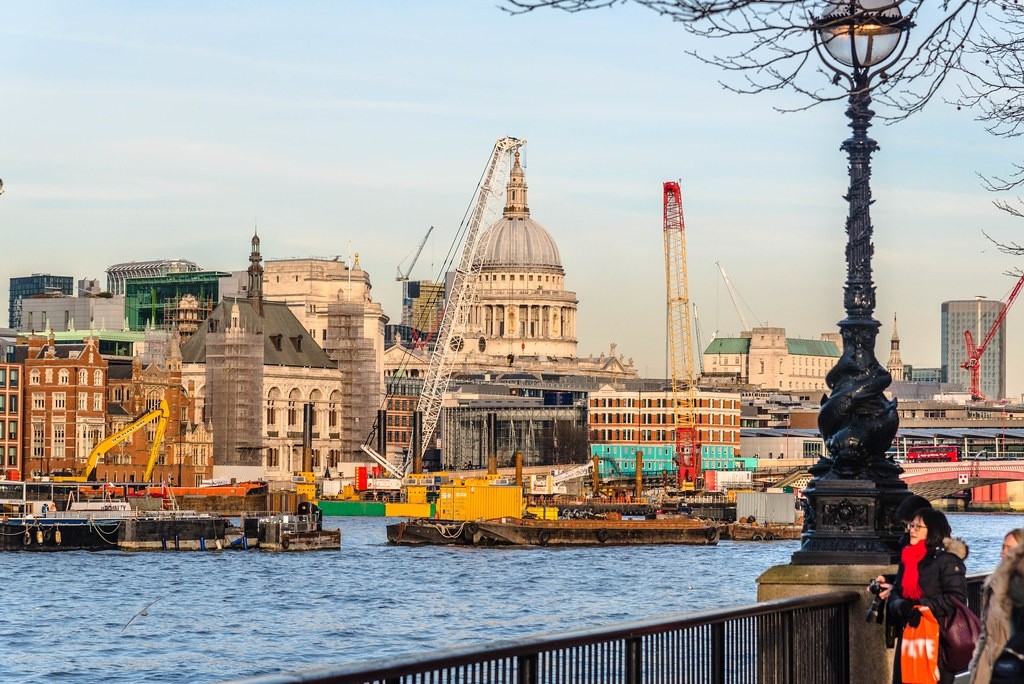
[908,446,962,463]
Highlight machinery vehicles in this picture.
[48,396,170,482]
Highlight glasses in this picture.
[907,522,927,531]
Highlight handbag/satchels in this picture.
[942,593,982,652]
[901,606,941,684]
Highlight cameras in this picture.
[865,579,886,625]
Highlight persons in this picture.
[42,501,49,518]
[967,528,1024,684]
[867,495,933,600]
[867,508,969,684]
[49,502,57,512]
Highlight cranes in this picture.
[395,226,435,342]
[960,276,1024,402]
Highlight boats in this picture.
[0,490,342,553]
[386,515,726,549]
[723,522,805,541]
